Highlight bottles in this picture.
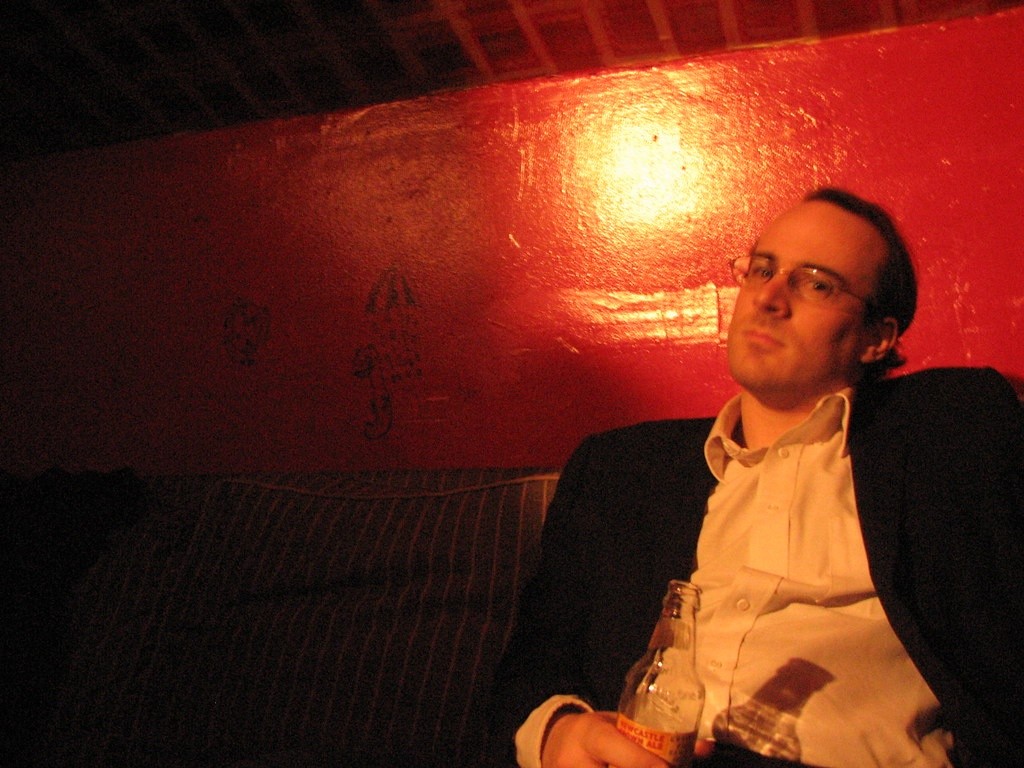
[600,579,705,768]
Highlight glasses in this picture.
[730,254,863,301]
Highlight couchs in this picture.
[0,460,564,768]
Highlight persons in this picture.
[475,186,1023,768]
